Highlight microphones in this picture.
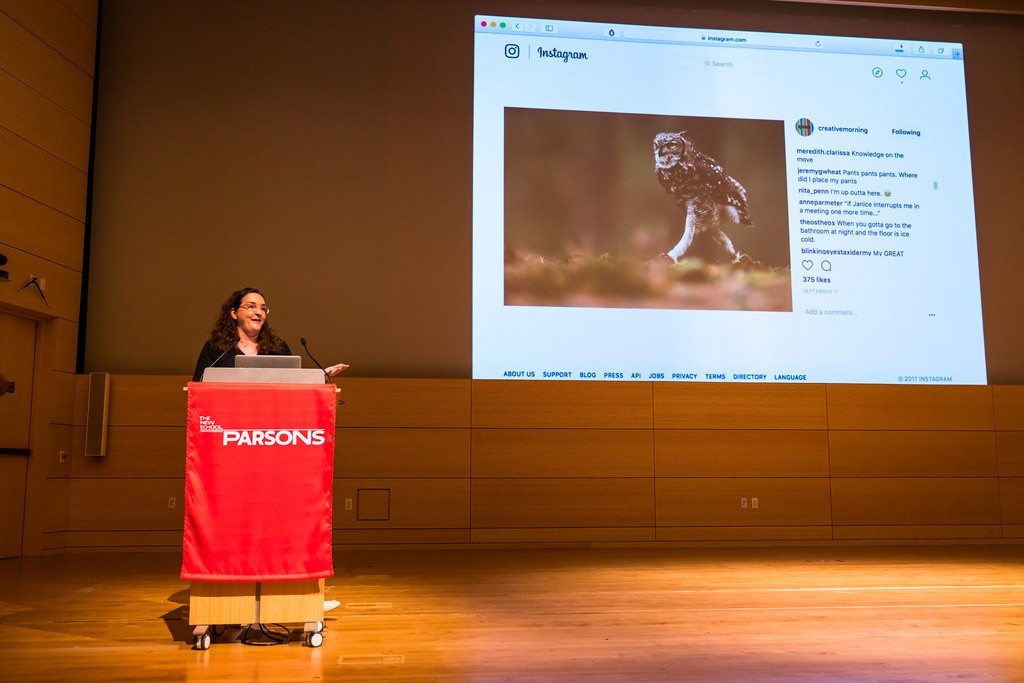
[301,337,333,384]
[200,336,241,381]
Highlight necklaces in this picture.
[240,339,258,347]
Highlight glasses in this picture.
[234,303,273,315]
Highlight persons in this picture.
[192,287,349,628]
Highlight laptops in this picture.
[235,355,302,368]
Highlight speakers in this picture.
[85,371,111,456]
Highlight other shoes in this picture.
[324,600,340,614]
